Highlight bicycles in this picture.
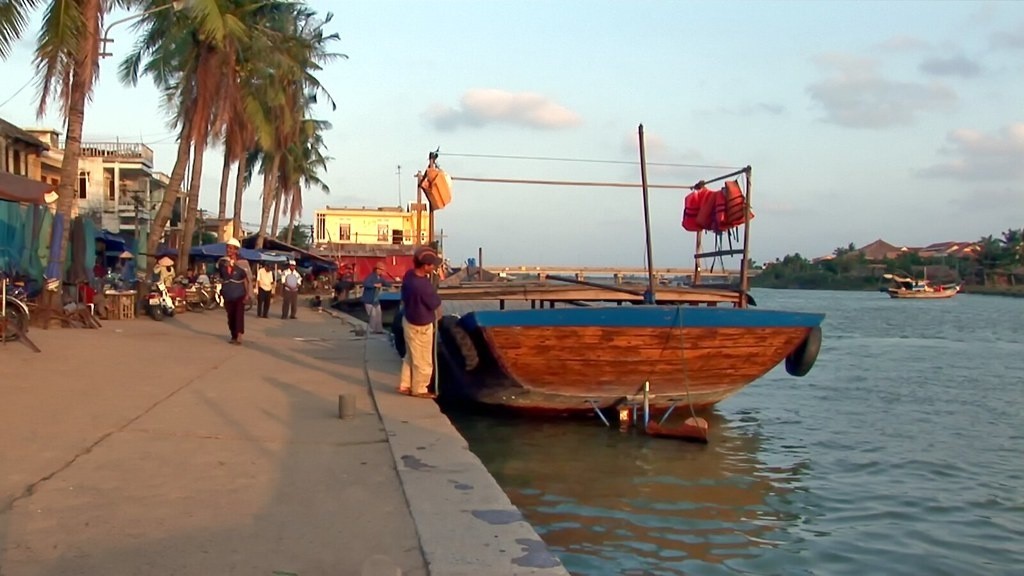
[0,295,30,343]
[145,270,332,322]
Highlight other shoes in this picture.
[370,330,386,335]
[237,337,242,344]
[411,391,436,398]
[398,387,411,395]
[229,339,236,343]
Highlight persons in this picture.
[96,238,321,344]
[363,262,395,333]
[397,247,443,399]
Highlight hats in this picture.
[288,260,296,266]
[158,256,174,266]
[374,262,384,270]
[226,238,240,248]
[119,251,134,258]
[414,246,442,267]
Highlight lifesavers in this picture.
[437,315,481,372]
[785,327,823,377]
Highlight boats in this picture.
[883,266,962,299]
[411,123,827,425]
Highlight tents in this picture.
[94,229,338,304]
